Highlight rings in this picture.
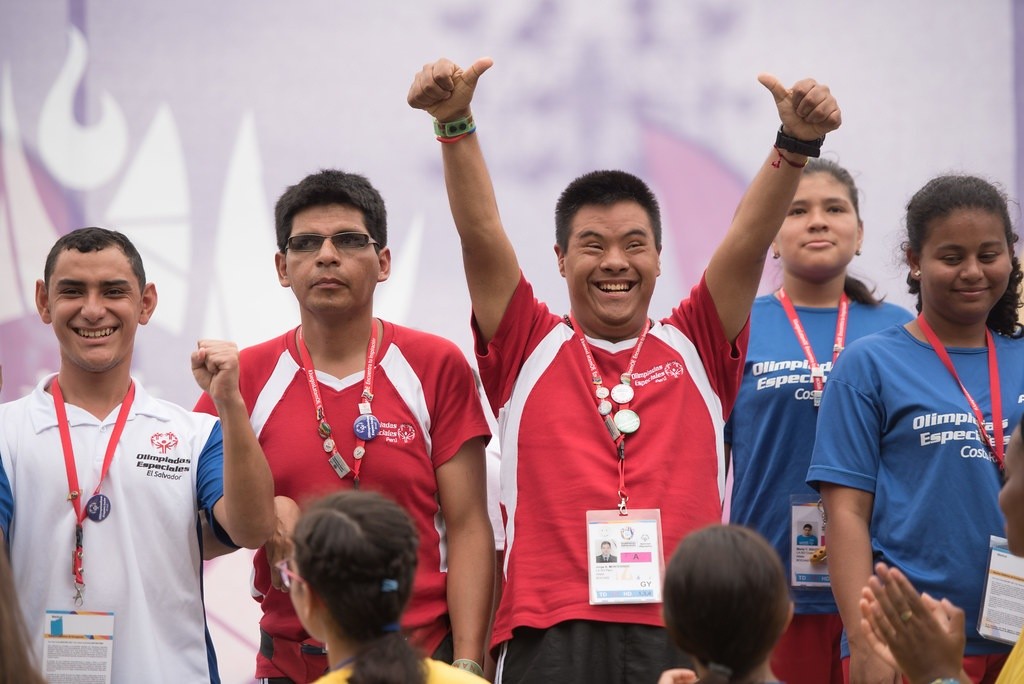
[900,610,913,622]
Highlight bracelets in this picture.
[434,115,476,146]
[771,124,825,169]
[930,678,961,684]
[451,659,485,679]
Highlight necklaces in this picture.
[334,655,359,670]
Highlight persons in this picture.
[797,524,819,546]
[409,58,841,684]
[659,157,1024,684]
[0,169,506,684]
[596,541,617,563]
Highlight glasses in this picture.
[275,558,303,589]
[283,231,380,252]
